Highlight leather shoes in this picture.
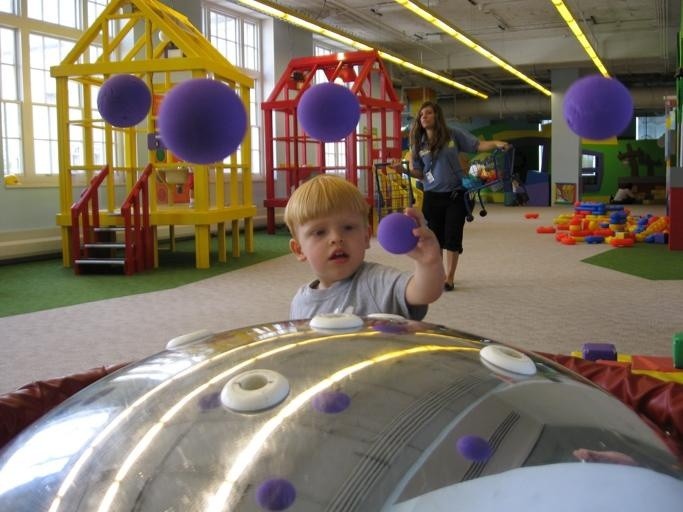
[443,282,453,291]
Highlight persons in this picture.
[388,100,513,292]
[572,447,636,466]
[611,183,642,204]
[280,175,444,322]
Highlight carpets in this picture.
[0,224,291,317]
[579,240,682,281]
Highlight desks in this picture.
[616,175,665,200]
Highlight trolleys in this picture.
[374,160,416,224]
[454,143,520,222]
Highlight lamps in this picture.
[239,0,613,98]
